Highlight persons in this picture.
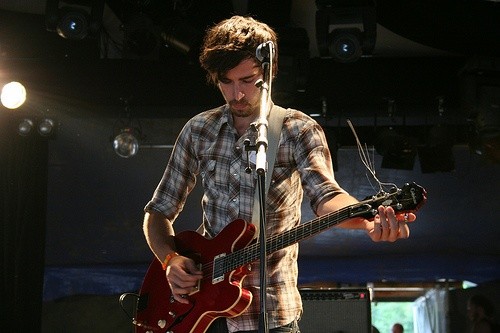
[142,15,417,333]
[392,295,494,333]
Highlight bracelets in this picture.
[162,253,179,270]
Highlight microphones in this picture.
[256,41,275,62]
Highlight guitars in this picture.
[134,181,428,333]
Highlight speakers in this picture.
[297,286,372,333]
